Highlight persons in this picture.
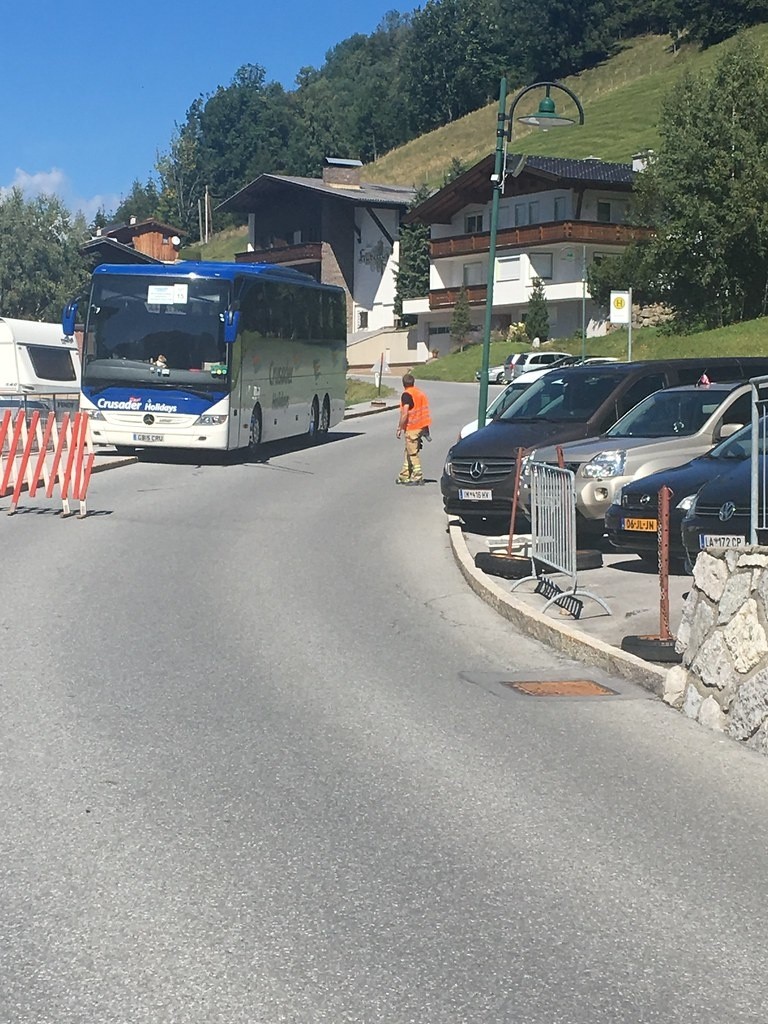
[396,374,431,486]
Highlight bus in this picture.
[62,260,347,462]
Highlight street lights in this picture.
[478,81,585,430]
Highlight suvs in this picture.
[517,375,768,523]
[458,366,568,441]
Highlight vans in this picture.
[440,356,768,537]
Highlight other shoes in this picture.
[395,478,410,484]
[406,480,424,486]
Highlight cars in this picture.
[503,354,521,383]
[603,414,768,563]
[680,454,768,576]
[548,356,601,368]
[513,352,571,379]
[575,358,618,366]
[475,365,511,385]
